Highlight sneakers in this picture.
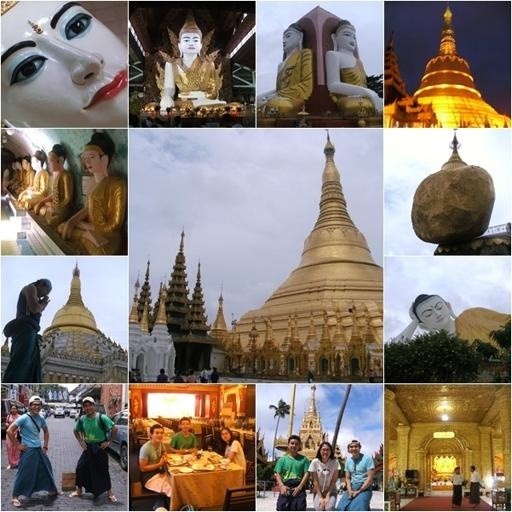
[69,490,83,497]
[47,492,65,496]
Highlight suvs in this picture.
[1,399,29,435]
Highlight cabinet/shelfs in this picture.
[384,474,422,511]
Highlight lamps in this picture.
[442,409,449,421]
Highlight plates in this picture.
[167,454,231,473]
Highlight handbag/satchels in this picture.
[3,316,22,338]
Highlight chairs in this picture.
[130,416,255,511]
[491,489,505,510]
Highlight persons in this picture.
[273,435,311,511]
[388,294,510,354]
[138,423,171,499]
[171,417,197,454]
[129,368,142,382]
[140,19,243,118]
[57,132,128,256]
[6,404,22,470]
[5,144,74,227]
[157,369,169,383]
[8,395,57,507]
[469,465,481,508]
[307,370,315,382]
[221,428,246,473]
[258,23,314,118]
[69,397,118,504]
[2,278,52,382]
[451,467,464,508]
[337,440,375,511]
[174,366,219,383]
[2,1,128,128]
[308,442,341,511]
[369,369,375,382]
[325,19,383,120]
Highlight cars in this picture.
[107,408,128,470]
[39,404,81,422]
[372,480,377,489]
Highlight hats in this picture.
[29,395,42,406]
[82,396,94,404]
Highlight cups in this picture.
[208,445,212,452]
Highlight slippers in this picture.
[107,494,118,503]
[11,498,22,507]
